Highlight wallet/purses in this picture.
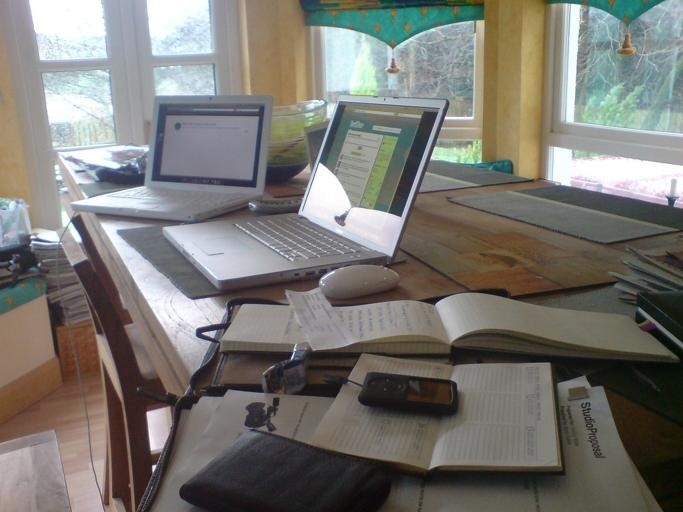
[179,429,392,512]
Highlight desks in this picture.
[287,161,683,298]
[55,146,468,505]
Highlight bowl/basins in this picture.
[264,98,329,186]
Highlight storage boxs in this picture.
[54,320,99,379]
[0,277,64,429]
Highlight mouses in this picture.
[320,265,400,298]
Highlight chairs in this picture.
[55,226,161,512]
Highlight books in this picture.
[309,354,566,476]
[220,292,680,367]
[30,225,92,326]
[607,245,683,305]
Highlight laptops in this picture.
[70,95,274,221]
[163,95,449,290]
